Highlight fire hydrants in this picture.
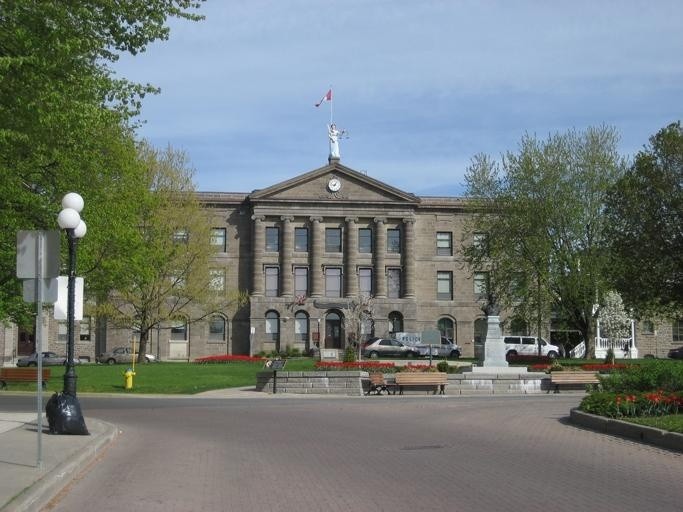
[122,367,136,390]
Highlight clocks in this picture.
[329,178,342,192]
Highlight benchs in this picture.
[0,367,51,390]
[396,372,448,394]
[551,370,601,394]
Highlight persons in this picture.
[328,123,345,156]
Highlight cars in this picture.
[358,333,562,363]
[94,347,156,366]
[15,350,82,369]
[667,344,682,360]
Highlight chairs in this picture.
[369,372,396,395]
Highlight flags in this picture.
[314,89,331,107]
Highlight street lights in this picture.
[315,317,320,349]
[54,191,88,401]
